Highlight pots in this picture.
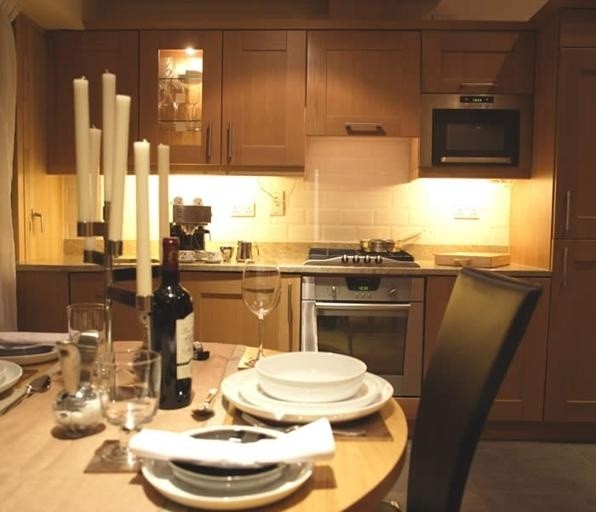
[361,232,421,253]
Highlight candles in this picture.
[101,69,115,204]
[134,138,153,296]
[73,76,88,222]
[157,143,170,263]
[89,126,101,222]
[109,95,131,241]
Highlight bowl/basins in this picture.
[159,56,172,77]
[179,74,204,86]
[256,352,368,400]
[184,70,201,74]
[169,430,288,490]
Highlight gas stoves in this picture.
[309,247,413,267]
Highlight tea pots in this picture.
[236,241,260,262]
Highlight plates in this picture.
[241,383,377,409]
[221,371,394,423]
[0,360,23,394]
[205,258,221,263]
[141,462,313,511]
[0,351,59,365]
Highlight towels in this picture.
[129,417,336,470]
[0,332,68,349]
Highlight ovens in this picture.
[302,276,422,398]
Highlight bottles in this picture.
[151,238,192,409]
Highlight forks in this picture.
[241,414,366,436]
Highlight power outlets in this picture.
[230,201,256,217]
[271,191,287,216]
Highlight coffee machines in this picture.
[169,205,211,261]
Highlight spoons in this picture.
[191,387,219,416]
[0,374,50,415]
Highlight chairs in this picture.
[406,266,545,511]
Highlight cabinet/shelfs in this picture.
[111,270,300,353]
[422,29,532,94]
[532,1,594,444]
[140,30,306,174]
[16,271,111,334]
[424,276,550,442]
[306,30,421,138]
[46,29,138,175]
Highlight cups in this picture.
[177,250,193,262]
[220,246,234,262]
[194,250,205,258]
[207,252,219,260]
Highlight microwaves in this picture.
[420,94,531,166]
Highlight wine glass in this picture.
[187,87,200,132]
[67,303,110,395]
[170,88,184,125]
[243,263,280,366]
[158,85,168,122]
[97,351,161,464]
